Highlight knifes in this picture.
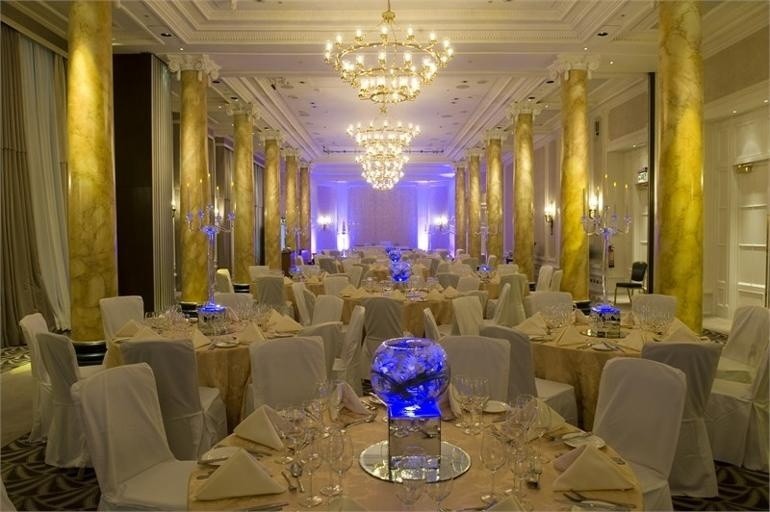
[243,500,290,512]
[553,432,593,444]
[207,339,216,350]
[601,340,618,351]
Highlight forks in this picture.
[562,486,638,511]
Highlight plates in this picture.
[210,340,243,347]
[590,343,616,352]
[562,430,608,450]
[202,447,244,470]
[569,497,621,511]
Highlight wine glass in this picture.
[273,374,549,511]
[539,298,669,339]
[139,302,272,340]
[358,276,441,299]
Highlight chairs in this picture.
[18,244,770,512]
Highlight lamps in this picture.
[324,0,455,105]
[346,104,424,158]
[354,149,409,192]
